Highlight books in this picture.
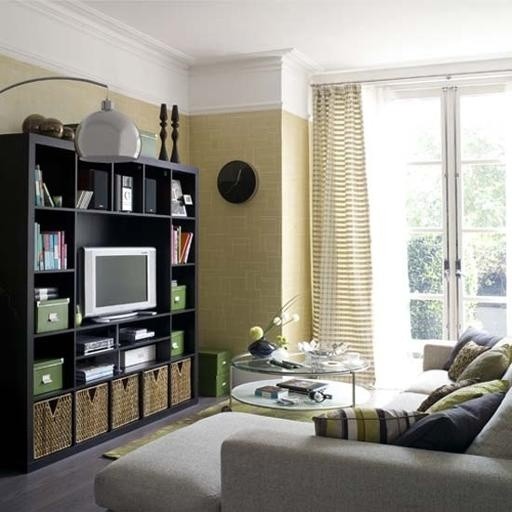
[75,190,94,210]
[34,163,68,272]
[76,371,114,383]
[172,223,194,266]
[255,383,289,400]
[76,363,114,378]
[274,377,330,395]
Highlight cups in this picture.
[346,352,359,364]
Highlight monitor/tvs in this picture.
[79,246,158,323]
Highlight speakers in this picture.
[145,178,158,214]
[81,168,109,210]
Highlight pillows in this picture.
[314,326,512,453]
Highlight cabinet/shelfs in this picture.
[0,134,200,475]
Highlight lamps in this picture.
[0,76,142,164]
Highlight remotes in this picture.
[271,359,294,369]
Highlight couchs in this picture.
[95,339,512,512]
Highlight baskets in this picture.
[142,365,169,417]
[170,358,192,407]
[75,383,108,442]
[111,374,140,430]
[34,393,72,459]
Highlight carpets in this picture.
[103,391,395,458]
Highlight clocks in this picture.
[217,159,259,205]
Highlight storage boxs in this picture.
[197,349,230,376]
[198,372,229,397]
[32,357,64,395]
[171,330,185,356]
[171,286,187,311]
[34,298,70,334]
